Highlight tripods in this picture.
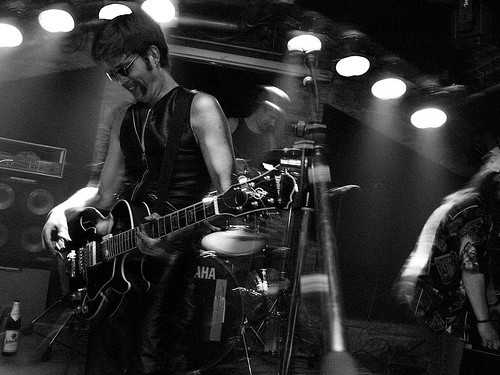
[247,198,318,355]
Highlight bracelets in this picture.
[477,318,491,324]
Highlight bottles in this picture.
[2,301,21,356]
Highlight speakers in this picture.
[0,168,64,270]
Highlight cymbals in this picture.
[260,148,302,158]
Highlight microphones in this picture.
[303,50,319,69]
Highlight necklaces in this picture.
[132,108,151,155]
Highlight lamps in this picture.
[0,0,448,130]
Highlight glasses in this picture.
[105,53,140,81]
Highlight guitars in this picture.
[64,163,298,322]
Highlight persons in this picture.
[41,12,241,375]
[392,147,500,375]
[227,85,292,172]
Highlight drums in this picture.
[198,207,268,259]
[241,247,293,296]
[176,245,244,375]
